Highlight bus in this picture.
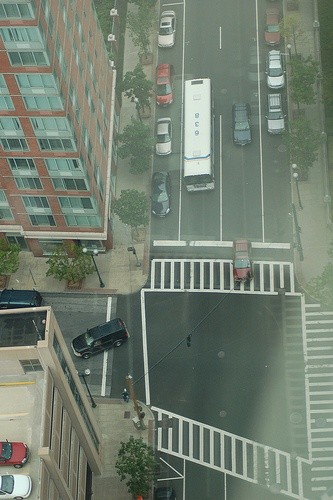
[184,78,215,194]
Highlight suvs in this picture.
[265,93,288,135]
[0,287,43,310]
[71,318,129,359]
[232,240,254,282]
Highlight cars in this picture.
[0,473,32,500]
[155,62,174,109]
[266,49,285,89]
[150,171,171,218]
[0,439,29,469]
[153,487,176,500]
[264,7,283,47]
[231,102,254,146]
[157,10,176,48]
[155,117,171,156]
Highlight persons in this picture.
[122,388,129,403]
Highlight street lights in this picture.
[82,248,104,287]
[73,369,96,409]
[292,164,303,211]
